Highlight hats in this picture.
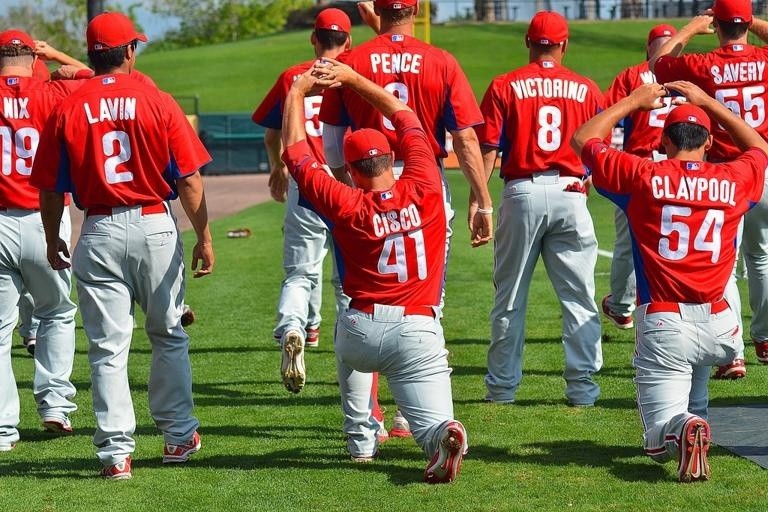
[87,12,147,50]
[714,0,752,23]
[376,0,416,9]
[659,104,710,154]
[0,31,35,50]
[345,128,391,173]
[646,24,678,44]
[528,11,569,45]
[315,8,351,33]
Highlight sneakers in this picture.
[677,416,710,482]
[44,417,72,432]
[350,410,412,461]
[755,340,768,362]
[102,455,132,481]
[280,328,320,394]
[163,431,201,463]
[714,359,746,379]
[602,295,634,329]
[424,422,465,483]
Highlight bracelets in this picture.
[476,207,494,214]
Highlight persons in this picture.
[250,0,381,393]
[467,11,613,408]
[646,1,768,379]
[603,24,687,328]
[319,0,495,444]
[570,80,767,482]
[281,56,469,483]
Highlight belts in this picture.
[646,302,729,313]
[88,202,166,215]
[351,300,435,316]
